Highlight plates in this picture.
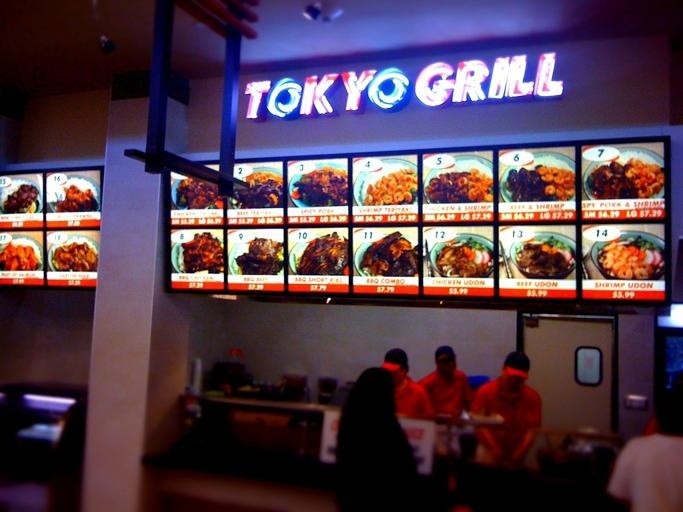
[352,158,419,206]
[498,150,575,203]
[169,160,350,275]
[422,153,495,205]
[46,234,99,272]
[580,146,665,200]
[48,173,100,213]
[0,177,44,214]
[0,234,44,272]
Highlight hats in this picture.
[435,345,455,365]
[380,349,407,373]
[501,351,530,381]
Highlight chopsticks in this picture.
[580,258,589,280]
[424,239,435,278]
[497,238,516,279]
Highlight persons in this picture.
[471,350,543,469]
[608,387,682,511]
[336,366,421,510]
[646,369,683,438]
[382,347,434,417]
[416,346,474,420]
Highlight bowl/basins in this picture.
[589,230,664,280]
[509,230,575,279]
[352,233,418,278]
[429,232,495,278]
